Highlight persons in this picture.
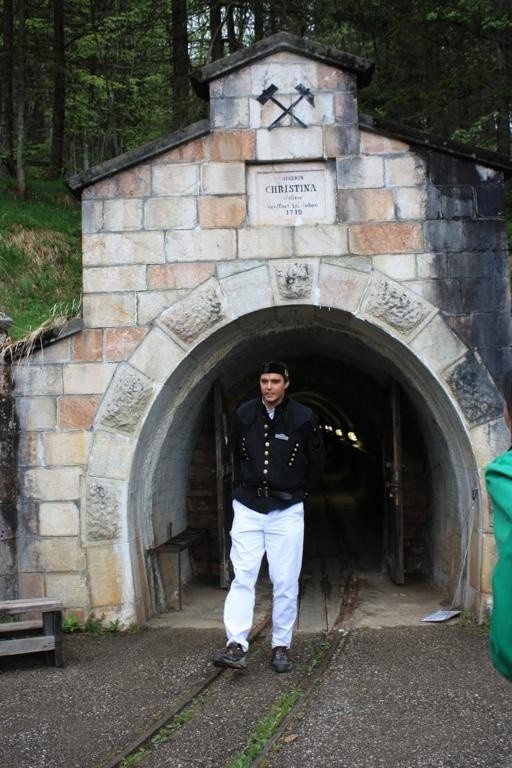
[484,368,511,684]
[210,357,327,672]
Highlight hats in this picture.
[257,355,290,381]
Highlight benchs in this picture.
[1,597,71,671]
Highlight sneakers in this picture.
[269,643,293,673]
[210,640,249,670]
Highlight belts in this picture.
[239,483,304,503]
